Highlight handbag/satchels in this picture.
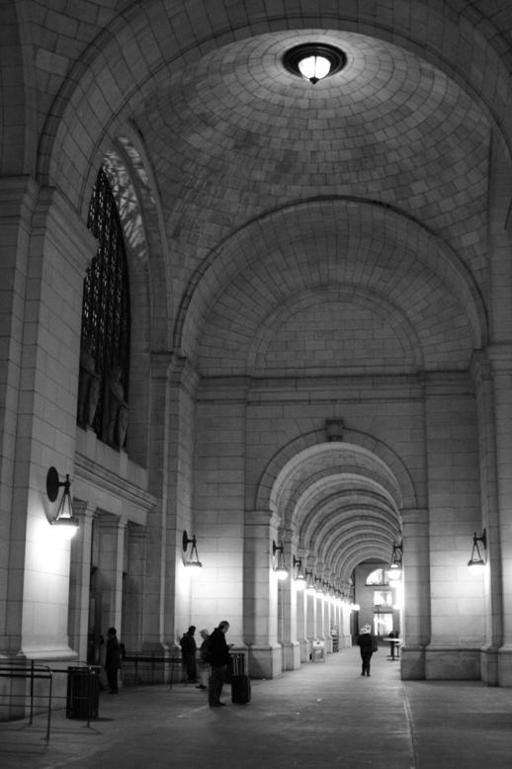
[200,636,212,662]
[371,637,378,652]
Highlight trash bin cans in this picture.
[67,666,97,718]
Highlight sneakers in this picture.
[209,702,223,707]
[196,685,206,689]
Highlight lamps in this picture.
[282,42,347,85]
[271,540,326,600]
[44,466,82,541]
[383,540,403,589]
[467,526,490,568]
[181,528,205,569]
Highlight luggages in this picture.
[231,653,250,705]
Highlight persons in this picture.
[357,628,377,676]
[179,625,200,683]
[205,620,235,707]
[195,629,212,689]
[105,627,121,694]
